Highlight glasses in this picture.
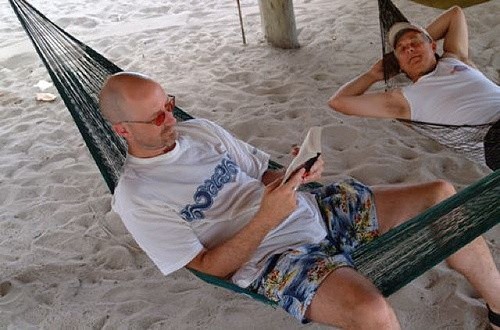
[120,95,177,126]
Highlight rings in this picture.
[293,187,297,191]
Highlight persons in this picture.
[331,6,500,173]
[94,70,500,330]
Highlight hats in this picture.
[388,22,434,53]
